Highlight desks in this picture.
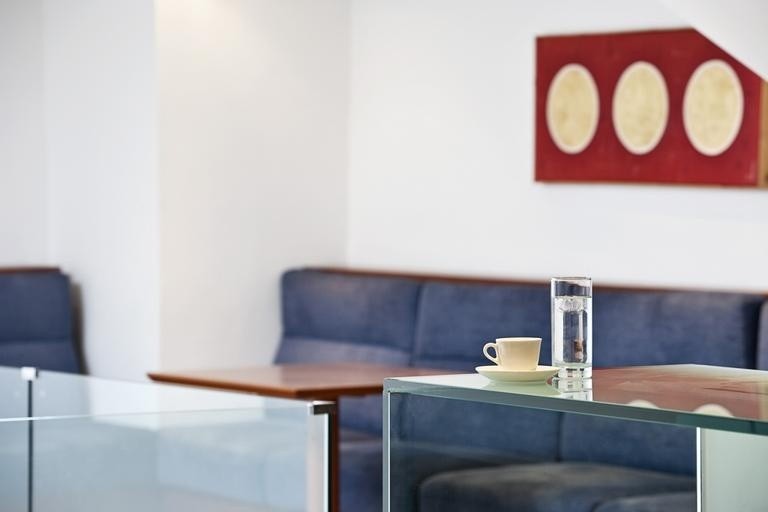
[145,363,472,511]
[381,362,767,511]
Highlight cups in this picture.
[549,277,594,382]
[483,336,542,369]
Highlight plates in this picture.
[475,365,564,383]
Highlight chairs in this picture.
[0,267,157,502]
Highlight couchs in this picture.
[158,263,767,510]
